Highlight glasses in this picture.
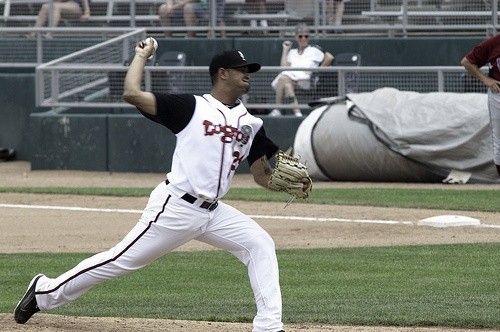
[297,35,308,39]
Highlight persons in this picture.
[245,0,268,27]
[461,33,500,175]
[158,0,227,38]
[269,25,335,117]
[21,0,91,37]
[12,36,313,332]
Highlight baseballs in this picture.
[145,37,159,51]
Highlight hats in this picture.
[209,50,261,74]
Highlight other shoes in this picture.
[294,112,302,116]
[269,109,281,115]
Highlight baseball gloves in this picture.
[268,151,313,199]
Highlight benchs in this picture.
[0,0,500,36]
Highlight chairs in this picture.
[125,51,187,67]
[331,52,362,98]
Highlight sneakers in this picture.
[12,273,45,324]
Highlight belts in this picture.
[165,179,218,212]
